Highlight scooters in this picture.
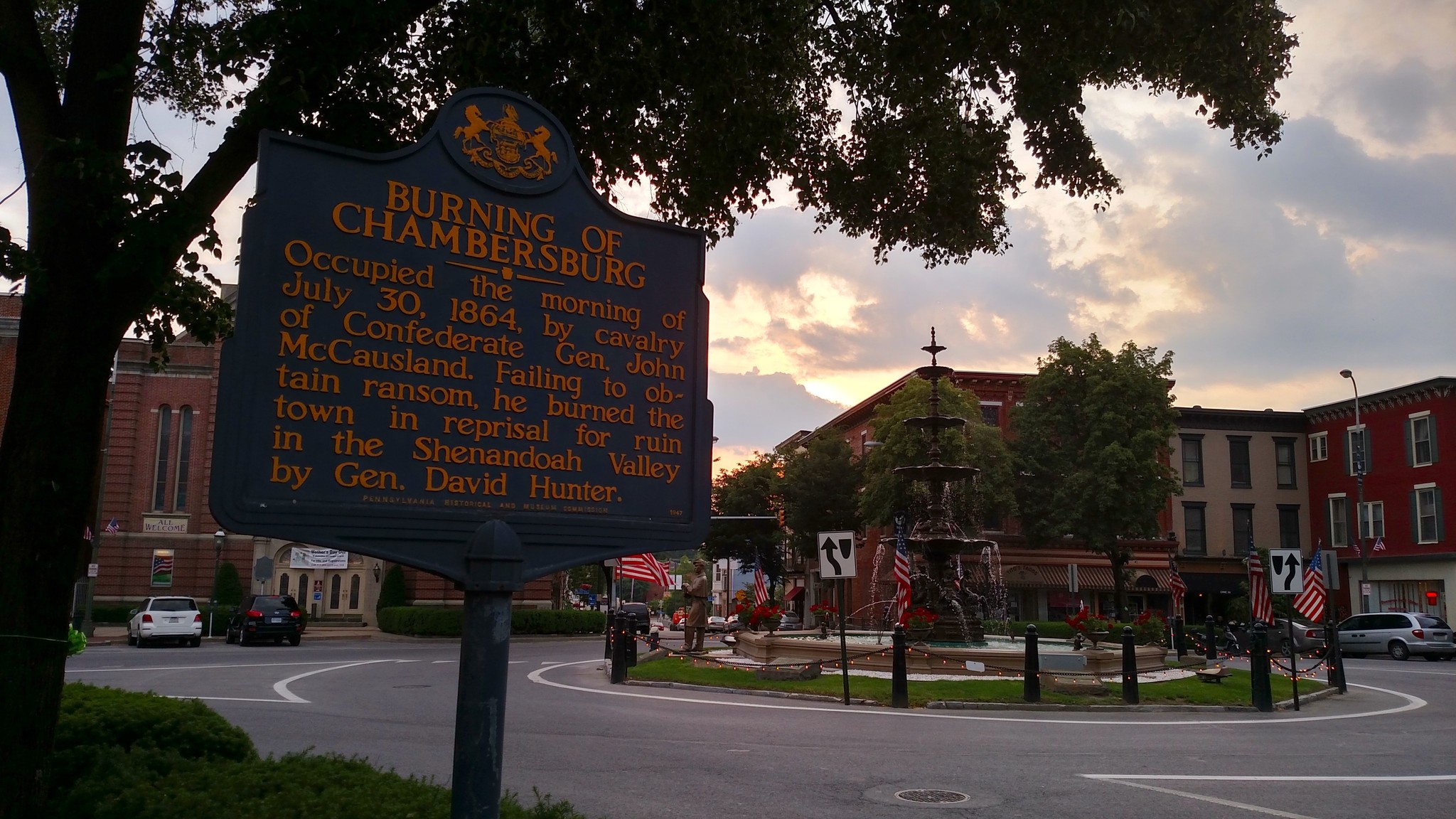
[1191,625,1241,656]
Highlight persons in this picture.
[680,557,709,652]
[1073,633,1086,651]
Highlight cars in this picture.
[1233,618,1327,658]
[704,616,726,634]
[759,611,803,631]
[676,618,686,631]
[651,622,664,631]
[723,614,744,632]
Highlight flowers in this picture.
[1078,616,1113,635]
[750,605,786,626]
[1064,606,1105,631]
[809,601,838,618]
[729,600,756,627]
[1133,609,1168,640]
[900,607,938,629]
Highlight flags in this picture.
[894,522,911,624]
[613,551,680,588]
[152,555,173,576]
[753,551,770,608]
[1372,538,1386,552]
[954,553,965,589]
[1349,536,1361,558]
[105,518,119,536]
[614,560,670,583]
[84,527,94,544]
[1248,526,1276,626]
[1167,554,1188,608]
[1292,547,1328,623]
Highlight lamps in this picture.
[373,563,381,583]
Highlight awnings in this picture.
[961,563,1188,591]
[783,585,805,602]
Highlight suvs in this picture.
[127,594,203,648]
[1315,612,1455,659]
[225,594,304,646]
[617,602,650,635]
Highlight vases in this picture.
[815,614,830,629]
[762,621,782,637]
[1082,631,1110,650]
[909,629,931,646]
[740,620,752,630]
[1144,633,1160,646]
[1081,631,1087,638]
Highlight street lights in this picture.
[212,529,225,634]
[1340,369,1369,612]
